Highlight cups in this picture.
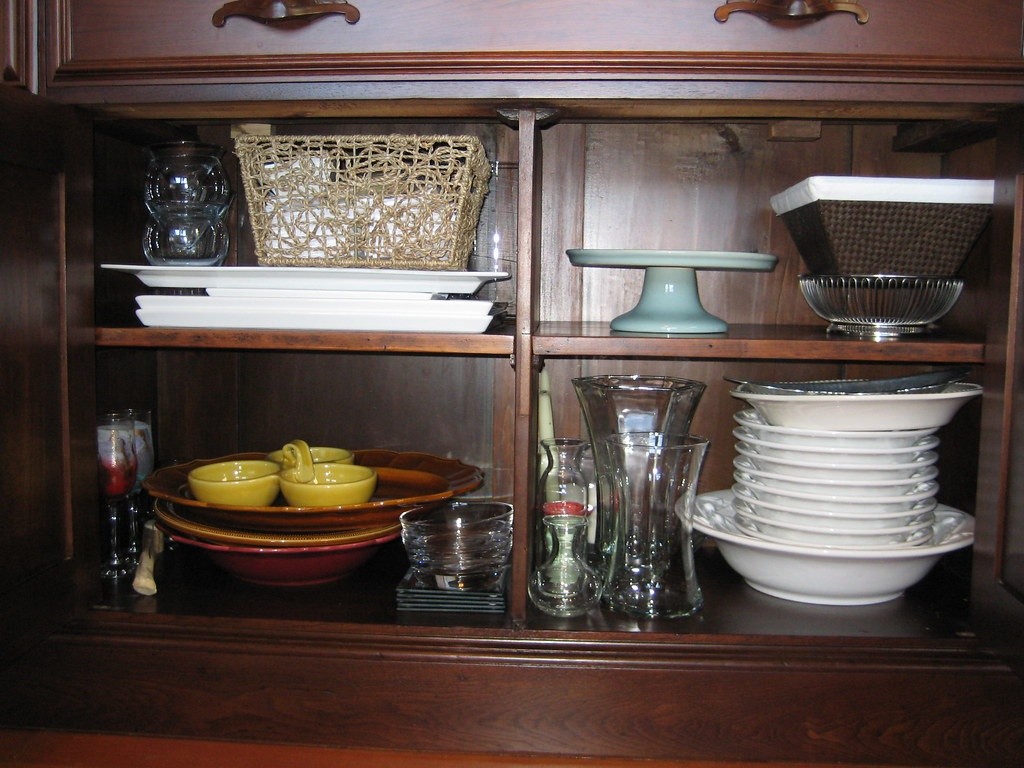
[572,366,712,620]
[269,437,380,507]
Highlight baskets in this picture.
[235,130,492,272]
[770,174,998,277]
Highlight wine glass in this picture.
[92,406,155,590]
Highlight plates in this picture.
[720,376,981,545]
[143,452,485,590]
[100,258,512,347]
[395,570,507,617]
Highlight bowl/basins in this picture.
[667,493,978,609]
[788,271,967,336]
[395,497,516,589]
[188,458,278,506]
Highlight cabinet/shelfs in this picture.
[0,0,1024,768]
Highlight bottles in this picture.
[533,435,598,621]
[132,138,229,265]
